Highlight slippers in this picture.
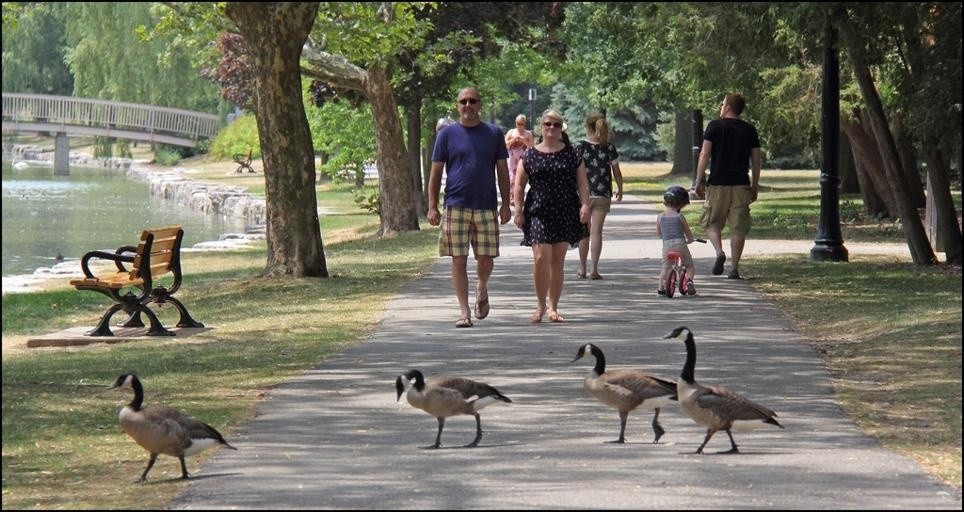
[530,305,547,323]
[474,283,490,320]
[547,311,565,323]
[455,318,473,328]
[590,273,603,280]
[577,272,587,279]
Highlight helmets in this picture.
[664,186,690,207]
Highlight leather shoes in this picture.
[729,273,740,279]
[713,251,726,275]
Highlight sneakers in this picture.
[687,278,696,294]
[657,288,667,298]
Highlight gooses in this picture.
[395,368,513,450]
[54,251,64,260]
[570,343,678,443]
[11,157,29,169]
[662,325,786,455]
[107,373,238,484]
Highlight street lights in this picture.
[528,86,538,130]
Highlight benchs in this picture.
[69,225,205,336]
[233,149,255,172]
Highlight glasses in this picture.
[542,121,563,127]
[457,98,481,104]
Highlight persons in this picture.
[503,113,535,207]
[694,92,761,280]
[435,110,456,135]
[571,113,623,280]
[511,108,590,323]
[653,186,697,298]
[427,86,509,328]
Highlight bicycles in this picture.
[663,238,708,299]
